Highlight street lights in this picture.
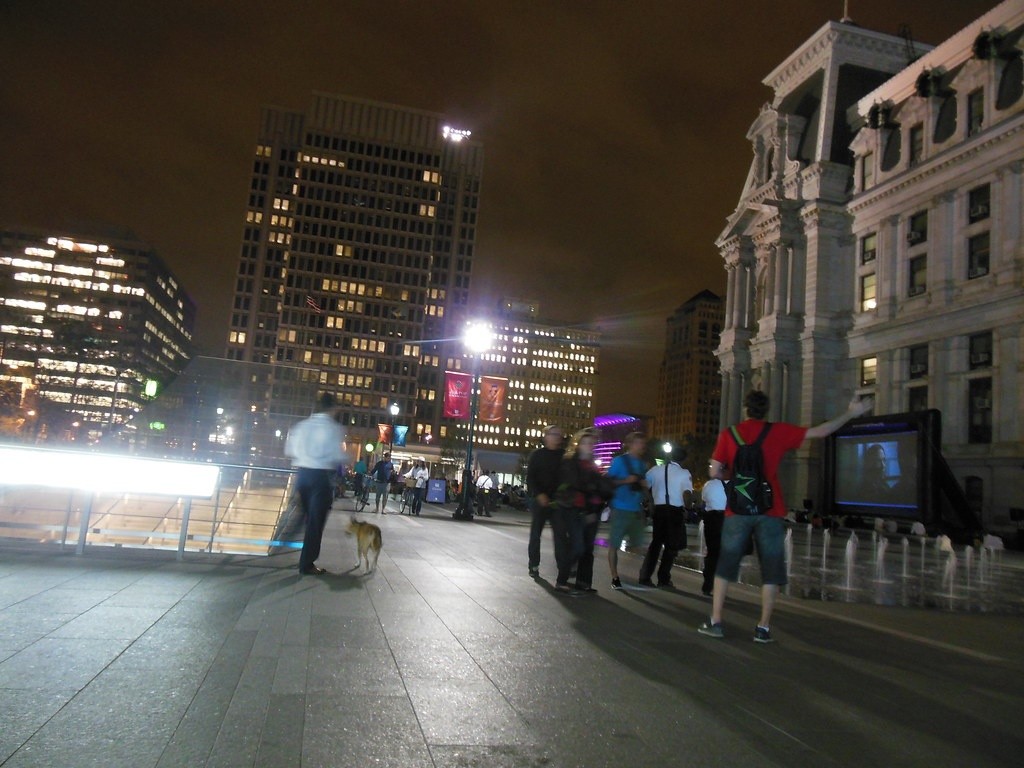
[449,320,494,522]
[390,403,400,453]
[215,407,224,442]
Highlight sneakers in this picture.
[697,617,724,637]
[555,583,585,598]
[577,586,598,594]
[611,577,623,590]
[754,624,774,642]
[657,581,676,592]
[640,579,657,589]
[529,567,540,577]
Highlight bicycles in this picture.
[356,473,384,512]
[400,474,425,516]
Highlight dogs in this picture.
[344,517,383,575]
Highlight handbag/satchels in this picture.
[665,512,687,549]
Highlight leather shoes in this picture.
[300,566,327,576]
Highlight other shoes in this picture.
[412,508,416,513]
[417,511,419,517]
[478,512,482,516]
[373,509,379,513]
[487,514,492,517]
[382,511,387,515]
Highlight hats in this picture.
[418,457,425,461]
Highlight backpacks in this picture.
[721,421,773,516]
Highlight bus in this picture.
[391,454,481,501]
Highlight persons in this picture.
[475,469,493,517]
[353,457,368,496]
[524,424,576,579]
[850,445,891,505]
[554,430,610,591]
[366,453,396,514]
[604,432,651,591]
[643,448,694,590]
[696,389,874,643]
[402,455,429,516]
[489,471,499,512]
[282,393,345,575]
[700,470,728,595]
